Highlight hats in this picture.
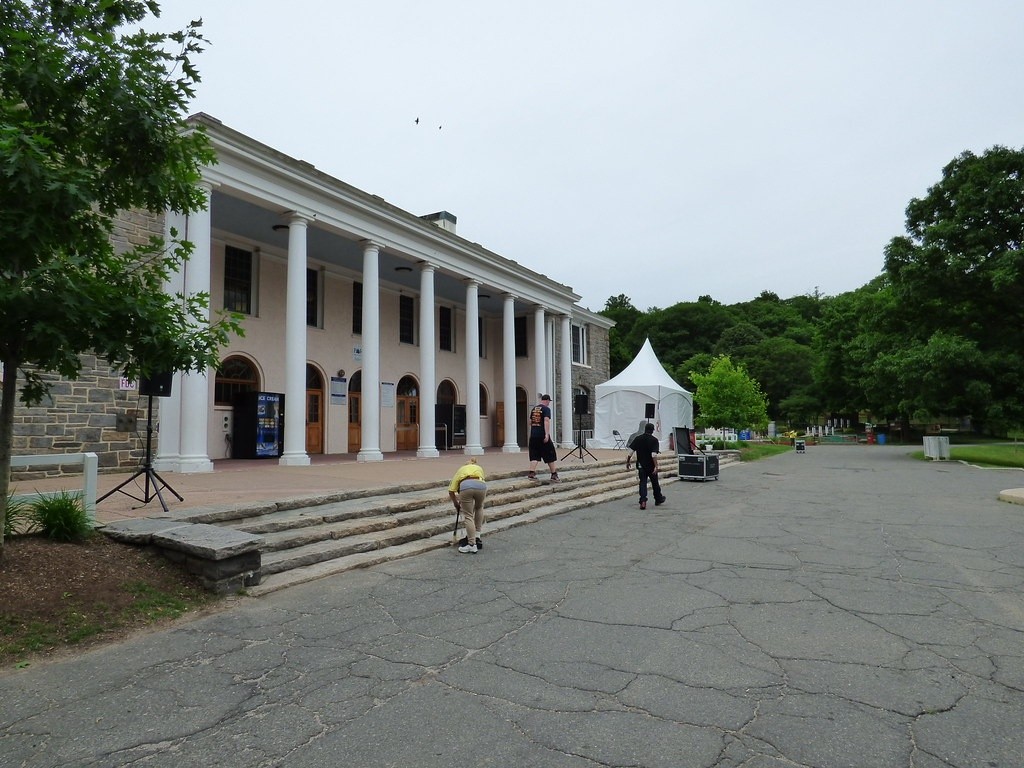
[645,423,655,430]
[542,395,552,401]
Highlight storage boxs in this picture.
[672,427,719,482]
[795,439,806,454]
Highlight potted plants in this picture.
[704,441,713,450]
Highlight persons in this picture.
[527,394,564,483]
[625,422,667,510]
[789,429,798,448]
[448,456,488,554]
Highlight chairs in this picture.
[613,430,627,451]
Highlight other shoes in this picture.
[458,545,478,553]
[528,473,539,480]
[640,502,646,509]
[655,496,666,505]
[551,474,562,483]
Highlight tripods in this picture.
[561,414,597,463]
[94,395,183,513]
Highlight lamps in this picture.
[272,225,288,232]
[395,267,413,273]
[478,295,491,299]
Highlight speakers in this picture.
[139,365,172,397]
[645,403,655,418]
[575,395,588,414]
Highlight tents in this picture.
[585,337,695,452]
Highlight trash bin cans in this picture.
[739,432,751,440]
[877,434,886,444]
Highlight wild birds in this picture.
[438,125,442,130]
[414,117,420,125]
